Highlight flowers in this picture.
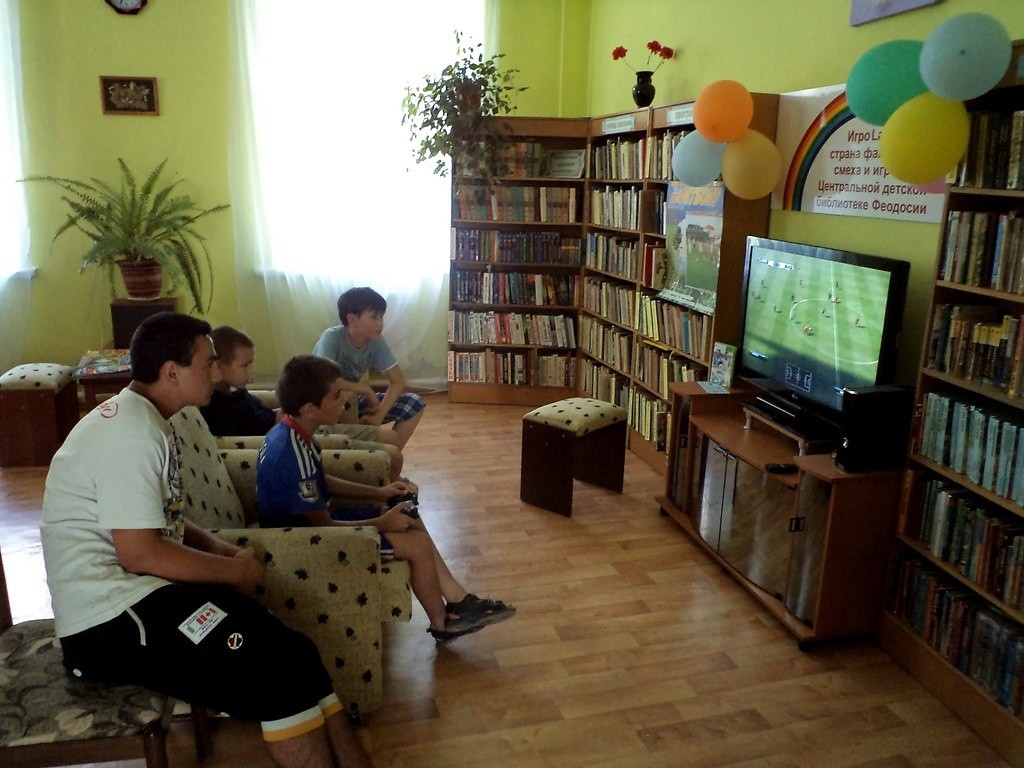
[613,41,674,71]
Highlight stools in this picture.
[520,398,629,518]
[0,363,80,469]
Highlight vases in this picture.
[632,71,655,108]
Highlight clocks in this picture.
[105,0,147,15]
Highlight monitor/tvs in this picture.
[736,236,911,431]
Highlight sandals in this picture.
[426,624,487,645]
[444,593,517,634]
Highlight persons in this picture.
[200,326,419,490]
[711,349,730,387]
[760,280,859,334]
[256,353,517,645]
[312,288,426,451]
[39,312,374,768]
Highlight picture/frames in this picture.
[99,75,160,117]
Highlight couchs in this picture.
[170,386,412,726]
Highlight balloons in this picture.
[672,79,782,199]
[845,13,1011,185]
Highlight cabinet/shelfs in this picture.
[448,39,1024,768]
[111,298,177,350]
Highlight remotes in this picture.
[766,464,798,475]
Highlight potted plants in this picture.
[398,31,530,200]
[15,157,232,316]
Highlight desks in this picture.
[78,349,134,413]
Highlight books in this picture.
[580,131,713,450]
[886,109,1024,722]
[447,142,587,388]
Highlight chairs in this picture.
[0,548,212,768]
